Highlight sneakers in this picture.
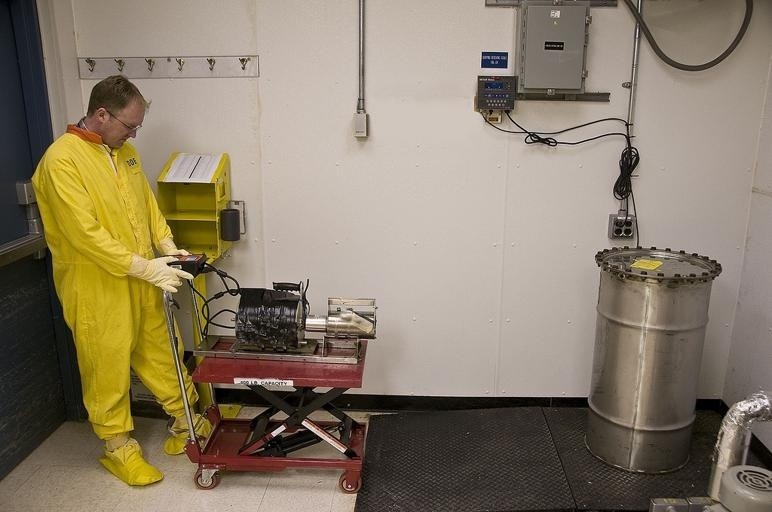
[163,413,213,455]
[101,437,165,487]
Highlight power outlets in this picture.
[608,214,636,239]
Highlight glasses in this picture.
[104,108,143,132]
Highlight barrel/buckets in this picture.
[585,256,715,473]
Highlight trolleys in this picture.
[161,255,377,495]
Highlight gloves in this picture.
[126,253,193,297]
[157,236,191,257]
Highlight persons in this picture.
[32,70,205,489]
